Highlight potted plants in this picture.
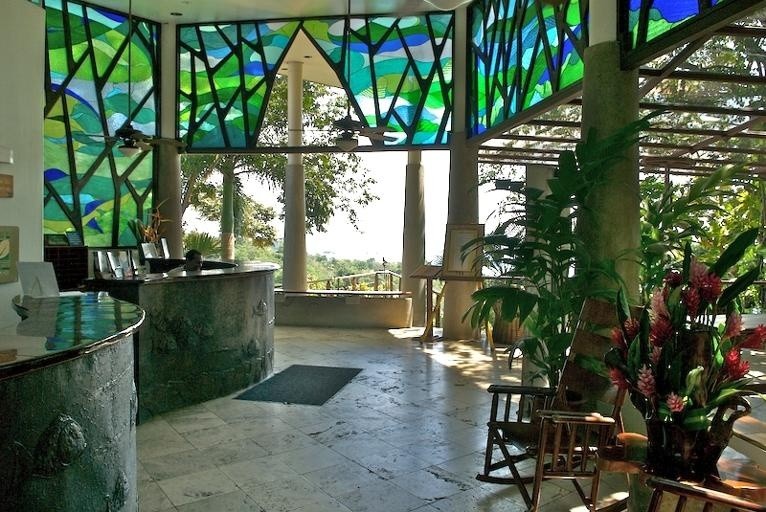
[457,103,759,435]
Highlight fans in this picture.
[76,0,188,152]
[286,1,397,143]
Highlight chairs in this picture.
[476,295,646,511]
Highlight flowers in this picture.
[568,225,766,436]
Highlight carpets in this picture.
[232,364,364,406]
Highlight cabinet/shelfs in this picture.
[44,245,88,292]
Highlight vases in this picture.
[627,386,753,483]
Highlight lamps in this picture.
[335,133,359,151]
[117,141,141,158]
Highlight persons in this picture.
[165,248,204,274]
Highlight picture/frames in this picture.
[44,231,84,247]
